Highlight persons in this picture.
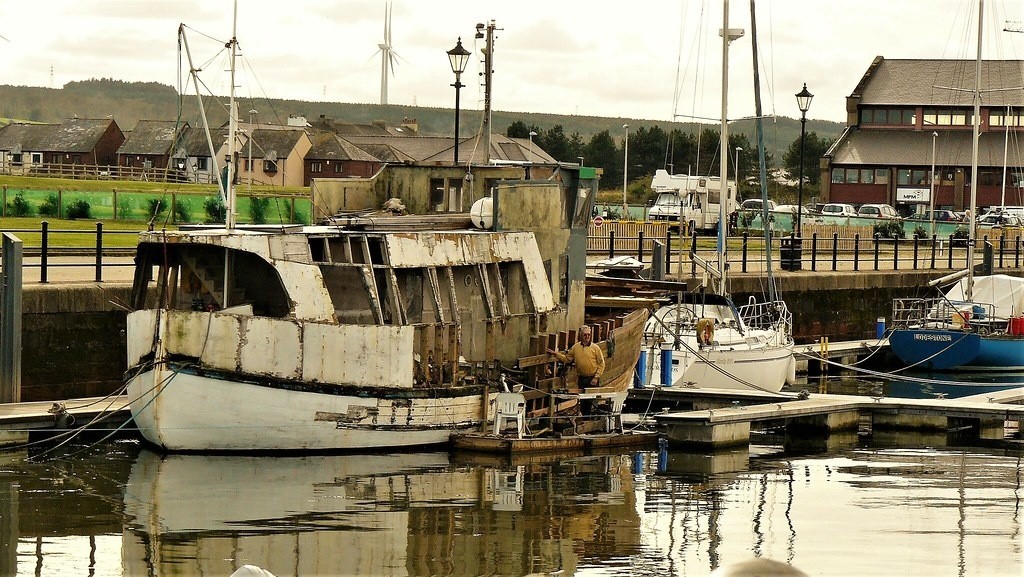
[994,211,1003,226]
[546,325,606,416]
[962,209,971,223]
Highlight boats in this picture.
[121,445,450,577]
[122,1,688,458]
[888,0,1023,374]
[622,1,795,409]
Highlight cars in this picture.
[769,205,816,224]
[805,203,1023,228]
[741,198,777,215]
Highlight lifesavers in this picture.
[697,317,714,348]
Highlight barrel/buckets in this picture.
[959,310,970,328]
[952,312,964,329]
[1019,316,1024,336]
[1007,317,1020,335]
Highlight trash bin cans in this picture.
[779,234,803,270]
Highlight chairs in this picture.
[493,389,529,440]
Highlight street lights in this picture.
[791,82,814,272]
[6,152,14,175]
[177,160,184,170]
[446,36,472,161]
[192,163,198,183]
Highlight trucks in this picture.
[650,169,736,236]
